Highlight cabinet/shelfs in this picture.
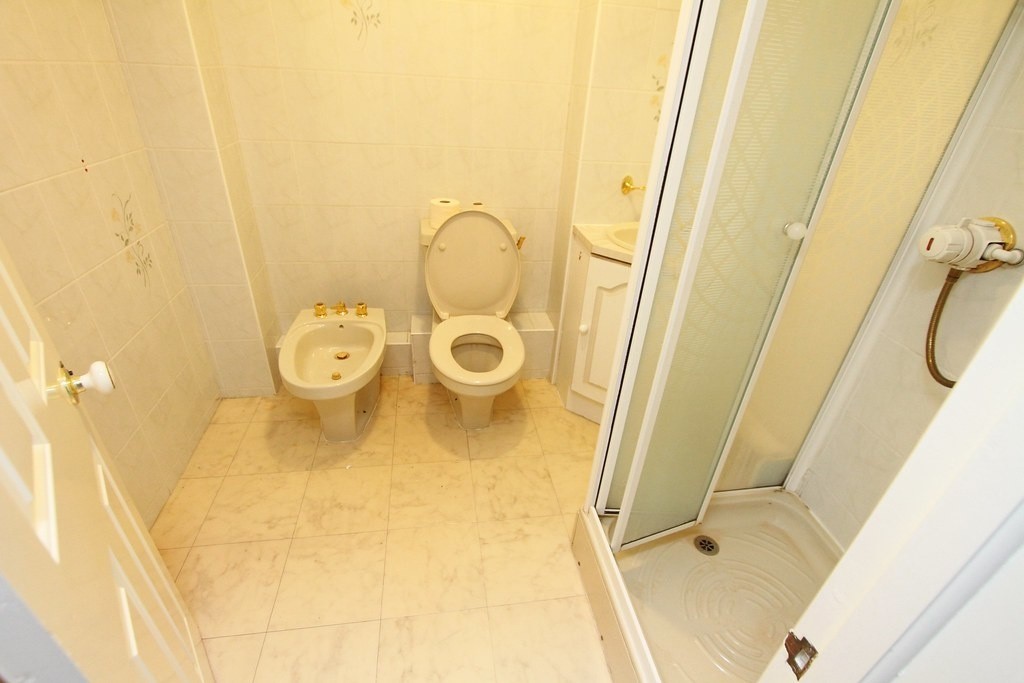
[554,235,633,426]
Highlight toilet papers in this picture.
[471,199,484,214]
[427,195,461,234]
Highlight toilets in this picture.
[416,216,533,430]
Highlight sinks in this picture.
[606,220,641,253]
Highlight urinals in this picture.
[277,298,392,445]
